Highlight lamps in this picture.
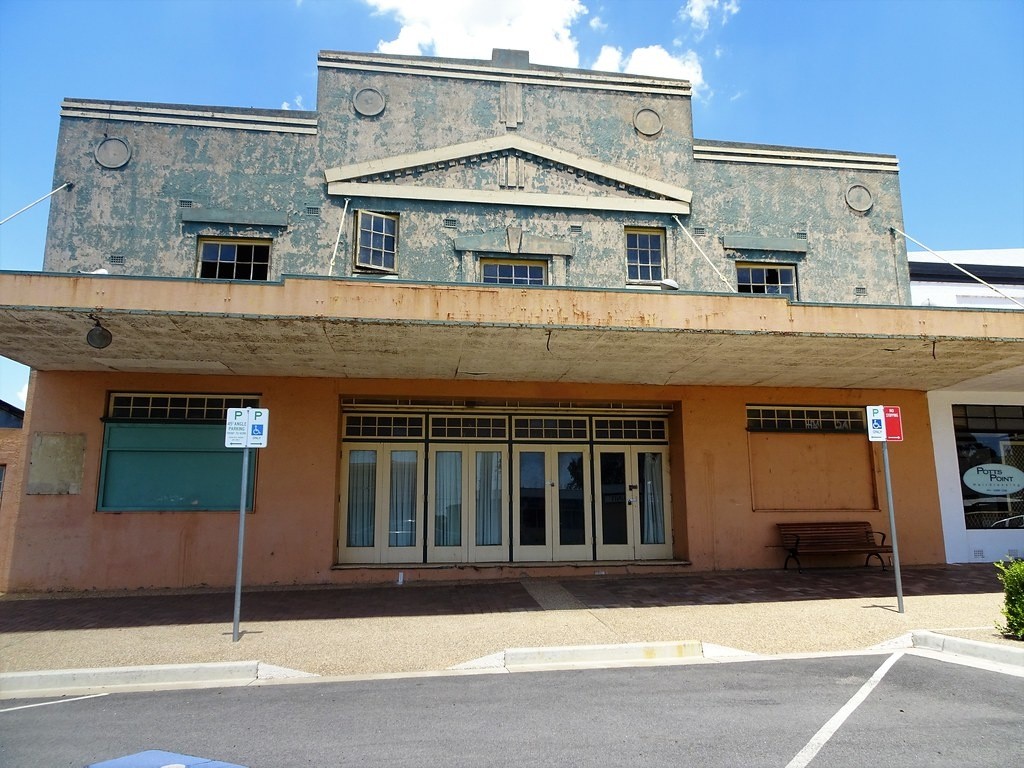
[86,313,112,348]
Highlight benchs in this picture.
[777,520,893,574]
